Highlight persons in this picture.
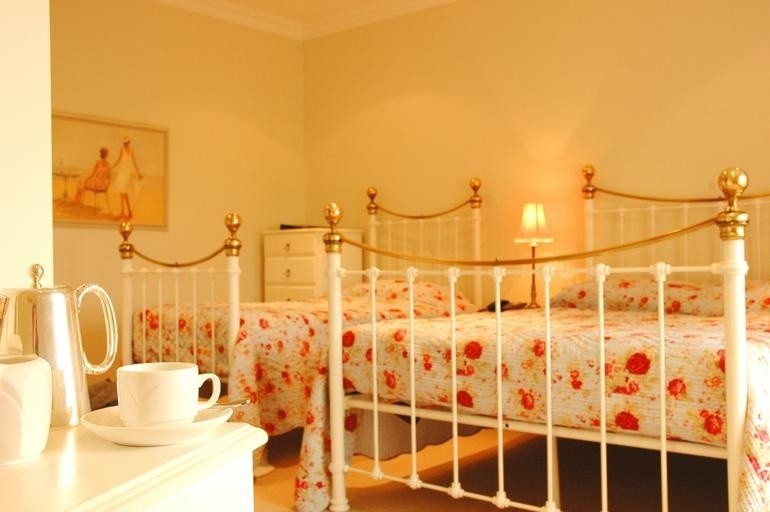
[109,137,145,219]
[73,146,111,208]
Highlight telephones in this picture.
[488,300,512,311]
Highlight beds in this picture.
[118,178,482,478]
[323,166,769,511]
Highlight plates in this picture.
[81,401,233,450]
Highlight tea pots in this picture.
[1,263,119,432]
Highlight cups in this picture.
[116,361,222,427]
[0,356,54,463]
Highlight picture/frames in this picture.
[52,110,172,233]
